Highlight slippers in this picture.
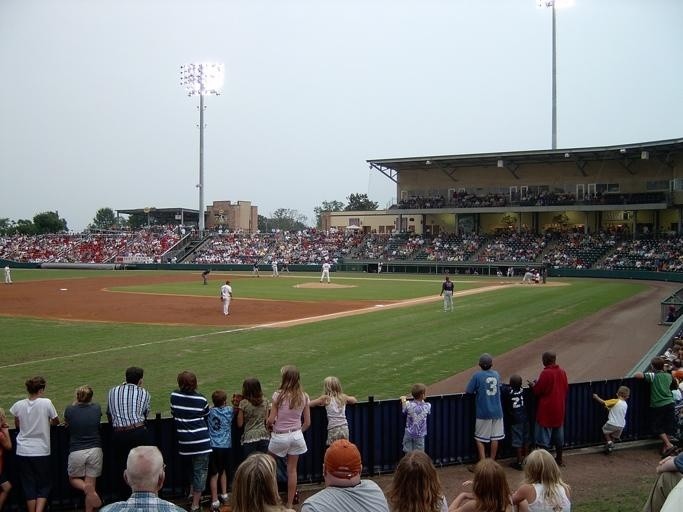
[661,445,676,458]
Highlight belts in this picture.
[273,427,301,434]
[114,422,145,431]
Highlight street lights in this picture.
[173,60,223,231]
[536,0,562,150]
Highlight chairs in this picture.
[387,190,671,209]
[359,230,682,273]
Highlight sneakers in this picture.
[82,483,101,507]
[605,442,614,455]
[187,494,229,512]
[468,464,476,472]
[508,463,524,471]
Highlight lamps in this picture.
[423,144,650,168]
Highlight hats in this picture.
[324,439,361,480]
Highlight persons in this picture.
[202,269,209,284]
[666,305,676,322]
[97,439,570,511]
[1,407,12,505]
[633,328,683,511]
[319,260,331,284]
[593,385,629,450]
[4,265,12,284]
[237,376,270,456]
[464,352,505,473]
[271,259,279,277]
[206,389,234,512]
[253,262,259,278]
[219,280,232,315]
[526,350,570,468]
[265,364,311,510]
[2,189,681,272]
[400,382,432,457]
[308,375,358,448]
[62,384,104,512]
[503,375,526,472]
[105,366,152,498]
[169,371,210,512]
[496,265,546,284]
[9,375,60,512]
[439,276,454,312]
